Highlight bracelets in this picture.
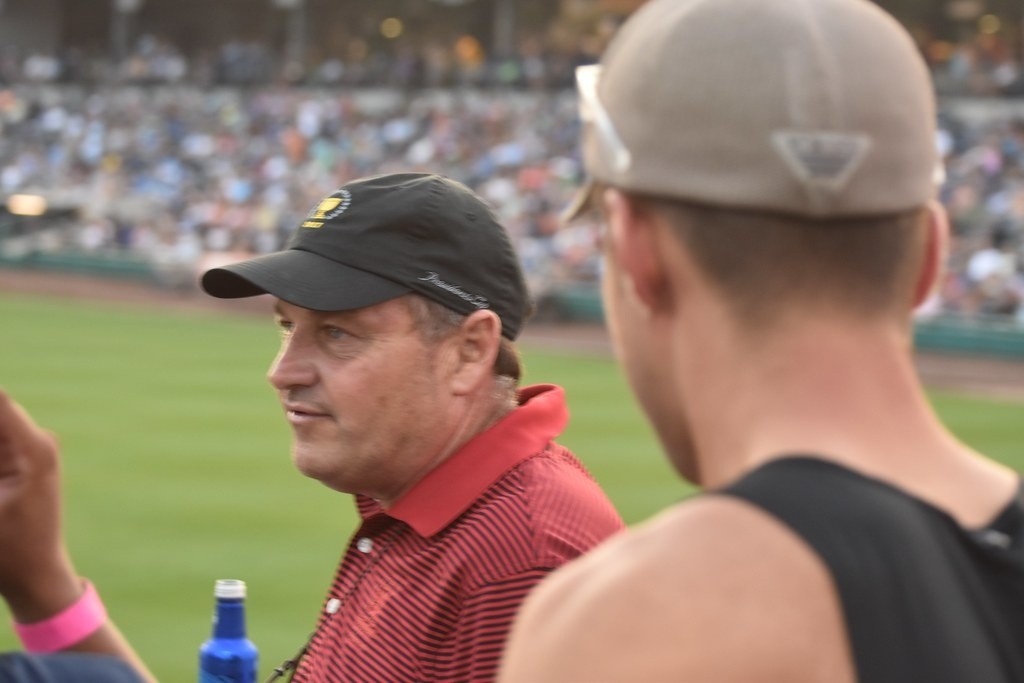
[11,578,107,652]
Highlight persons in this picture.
[0,394,158,683]
[201,173,624,682]
[495,0,1024,682]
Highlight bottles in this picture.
[197,579,259,683]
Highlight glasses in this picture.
[573,62,634,173]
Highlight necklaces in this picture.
[267,523,407,682]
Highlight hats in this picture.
[560,2,939,225]
[199,171,527,339]
